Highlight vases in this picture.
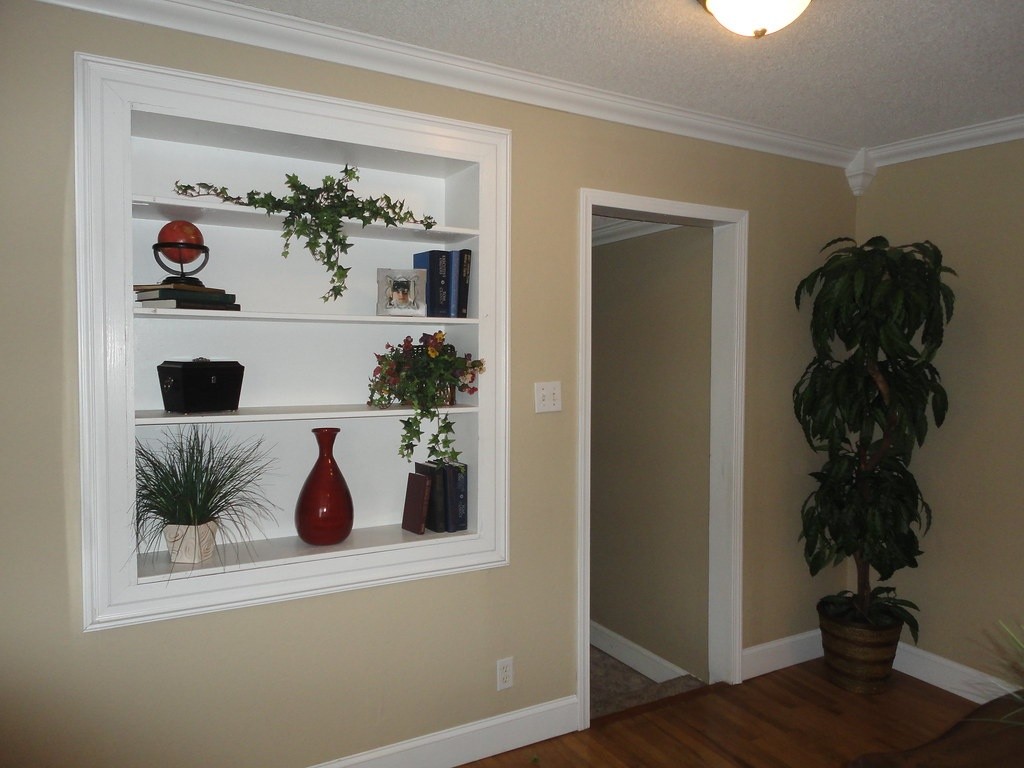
[296,427,353,544]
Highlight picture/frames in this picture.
[376,265,427,318]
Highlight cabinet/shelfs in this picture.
[70,48,511,630]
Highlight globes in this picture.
[152,221,210,286]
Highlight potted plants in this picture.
[793,231,960,690]
[128,422,287,587]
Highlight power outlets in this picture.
[534,381,562,414]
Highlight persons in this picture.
[392,282,412,309]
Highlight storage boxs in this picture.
[156,357,245,415]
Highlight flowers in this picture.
[368,329,488,474]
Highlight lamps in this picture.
[698,0,813,37]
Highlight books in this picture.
[401,457,468,535]
[414,249,472,317]
[134,283,240,311]
